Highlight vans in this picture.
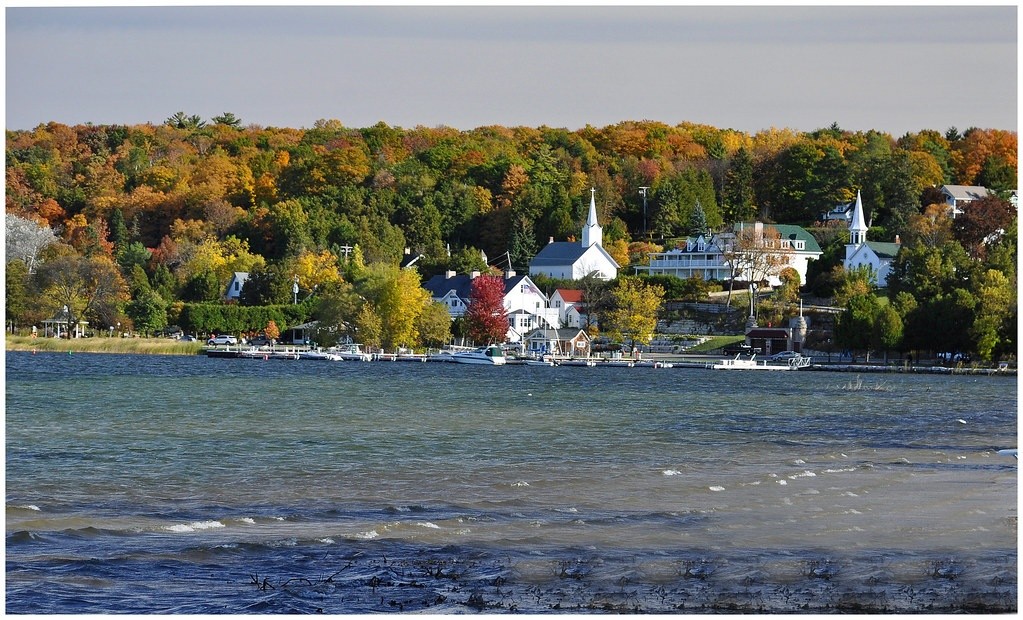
[505,341,526,352]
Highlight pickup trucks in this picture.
[723,342,761,356]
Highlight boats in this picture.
[526,361,554,367]
[450,344,506,366]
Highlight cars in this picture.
[771,351,801,361]
[207,335,237,345]
[937,352,969,361]
[248,335,277,346]
